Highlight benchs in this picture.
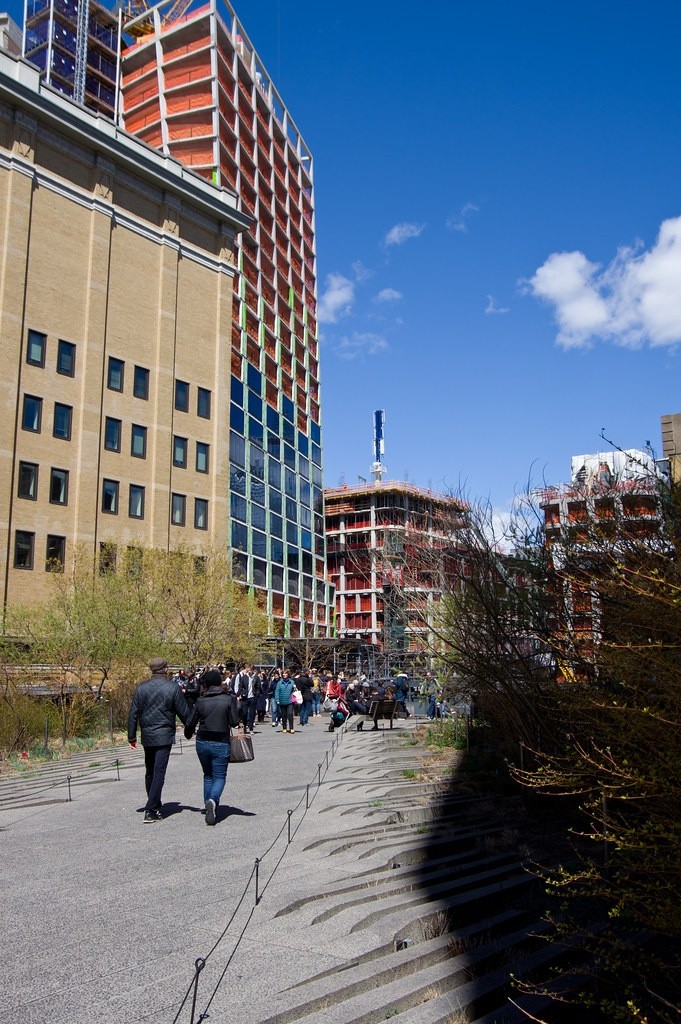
[368,700,404,733]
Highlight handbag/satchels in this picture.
[323,699,338,711]
[228,723,255,762]
[291,687,303,705]
[336,701,349,715]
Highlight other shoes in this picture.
[313,713,322,717]
[277,720,281,725]
[300,721,310,725]
[249,730,254,734]
[154,808,162,816]
[283,728,287,733]
[142,810,162,823]
[290,728,295,733]
[329,726,335,732]
[272,721,276,727]
[205,799,216,825]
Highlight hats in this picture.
[149,658,169,671]
[205,670,221,689]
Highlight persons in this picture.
[266,668,322,727]
[214,661,269,735]
[421,673,443,720]
[391,671,412,719]
[276,670,300,734]
[365,681,396,730]
[184,668,239,826]
[127,658,191,823]
[296,668,315,726]
[170,667,205,710]
[320,670,369,715]
